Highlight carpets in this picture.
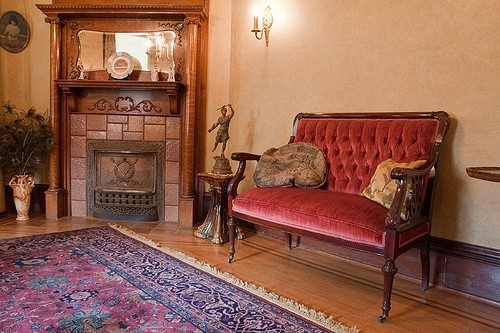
[0,222,361,333]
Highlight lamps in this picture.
[250,5,273,50]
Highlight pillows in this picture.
[364,158,425,220]
[253,142,326,188]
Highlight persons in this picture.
[4,14,21,46]
[208,103,234,157]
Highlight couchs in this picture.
[227,111,451,323]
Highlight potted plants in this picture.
[0,100,52,221]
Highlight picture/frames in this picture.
[0,11,30,54]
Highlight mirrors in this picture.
[76,30,176,83]
